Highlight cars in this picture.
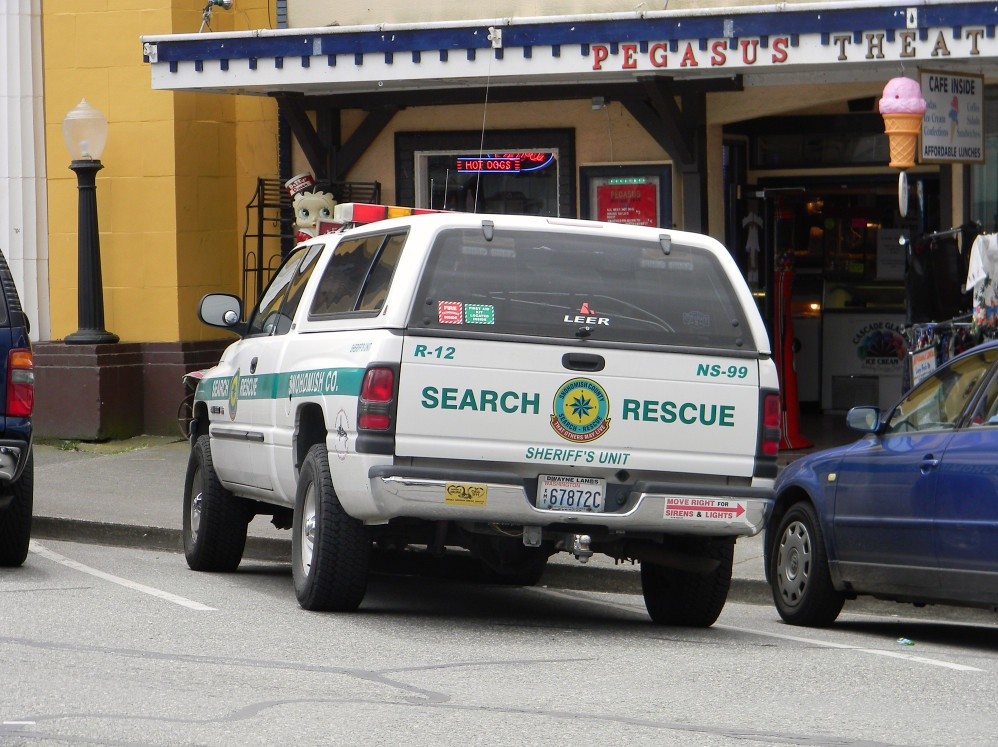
[758,341,996,627]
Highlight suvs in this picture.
[0,249,39,568]
[178,201,784,629]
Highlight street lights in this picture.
[62,98,124,346]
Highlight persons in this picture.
[293,185,340,241]
[942,376,988,428]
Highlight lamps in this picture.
[199,0,233,34]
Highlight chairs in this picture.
[442,238,705,301]
